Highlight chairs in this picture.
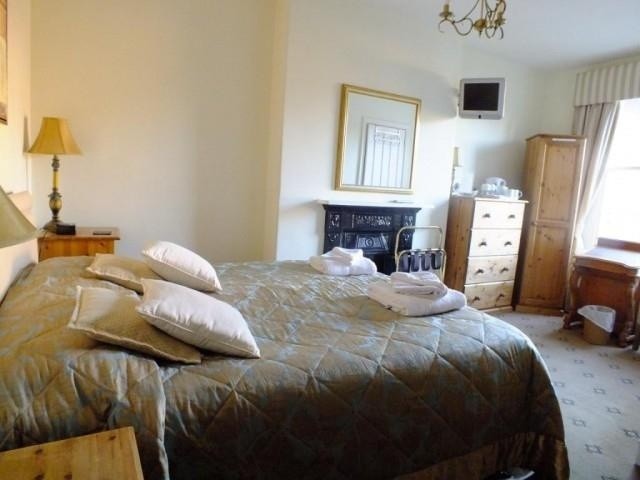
[393,226,454,284]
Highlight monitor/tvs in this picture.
[459,77,506,120]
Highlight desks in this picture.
[563,238,640,350]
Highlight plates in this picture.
[458,190,478,197]
[494,194,505,198]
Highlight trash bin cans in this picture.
[583,306,616,346]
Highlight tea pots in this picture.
[486,177,506,195]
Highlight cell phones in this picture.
[94,230,111,235]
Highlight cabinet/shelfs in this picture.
[324,204,420,275]
[443,191,529,314]
[516,133,586,318]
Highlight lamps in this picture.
[27,117,82,234]
[437,1,506,41]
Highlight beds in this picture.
[1,252,568,480]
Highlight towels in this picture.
[321,247,364,265]
[391,270,448,299]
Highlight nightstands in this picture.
[38,227,121,262]
[0,425,144,478]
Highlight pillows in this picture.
[139,278,254,356]
[89,247,162,292]
[66,284,203,364]
[142,235,217,293]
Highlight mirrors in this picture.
[334,83,422,194]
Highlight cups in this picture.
[488,184,497,197]
[510,189,522,200]
[482,184,488,197]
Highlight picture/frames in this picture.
[1,0,9,125]
